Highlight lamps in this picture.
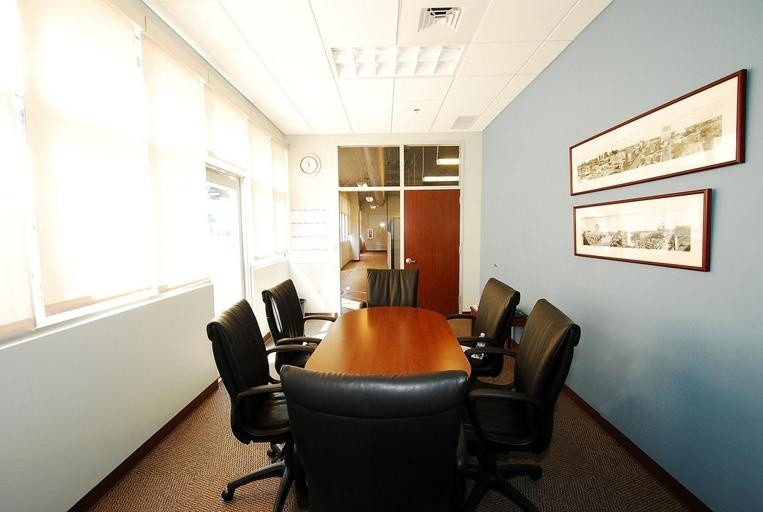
[420,147,459,183]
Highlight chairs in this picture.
[365,267,419,307]
[460,298,581,512]
[206,299,290,512]
[273,360,467,512]
[259,277,337,366]
[454,276,521,379]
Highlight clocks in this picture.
[298,154,319,177]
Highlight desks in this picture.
[303,306,471,380]
[468,304,527,350]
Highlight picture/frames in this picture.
[571,187,713,272]
[367,228,373,238]
[568,68,749,193]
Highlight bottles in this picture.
[474,333,485,360]
